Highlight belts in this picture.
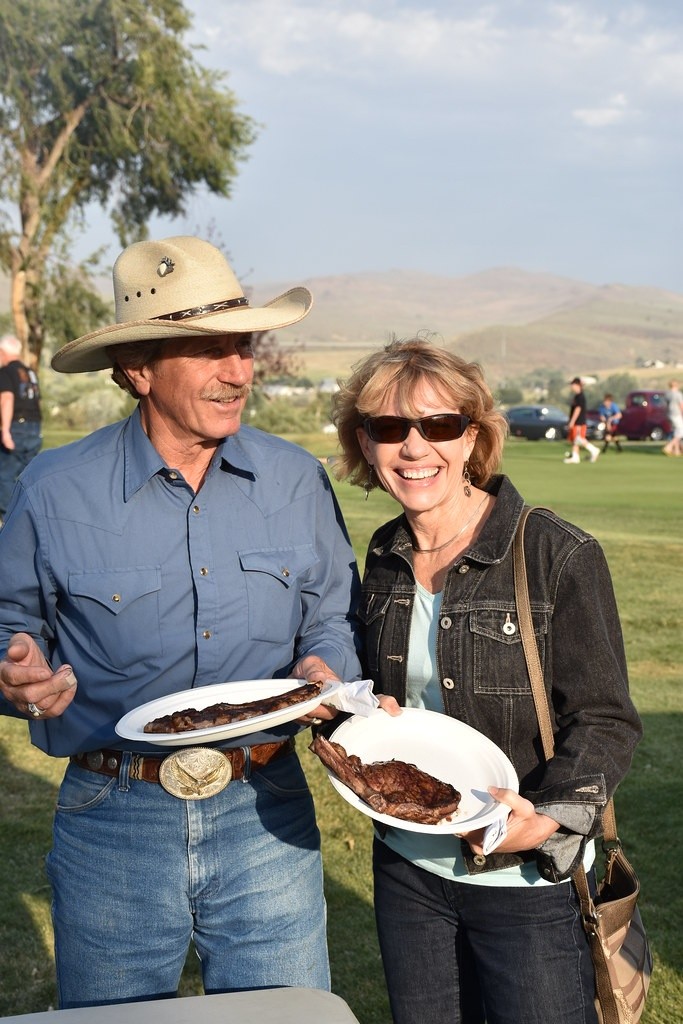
[63,736,298,798]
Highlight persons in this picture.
[308,337,642,1024]
[597,393,623,453]
[663,379,683,458]
[0,236,364,1007]
[0,333,43,524]
[563,378,600,464]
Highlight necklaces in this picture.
[412,492,491,553]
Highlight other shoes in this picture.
[563,455,580,464]
[591,445,602,463]
[662,445,683,458]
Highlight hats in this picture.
[50,236,316,372]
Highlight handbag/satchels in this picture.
[581,842,655,1024]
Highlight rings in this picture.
[27,703,46,716]
[311,717,322,725]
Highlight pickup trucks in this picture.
[583,390,674,442]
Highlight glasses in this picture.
[351,405,475,443]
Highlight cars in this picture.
[505,404,604,442]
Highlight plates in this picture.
[324,707,520,836]
[114,679,344,747]
